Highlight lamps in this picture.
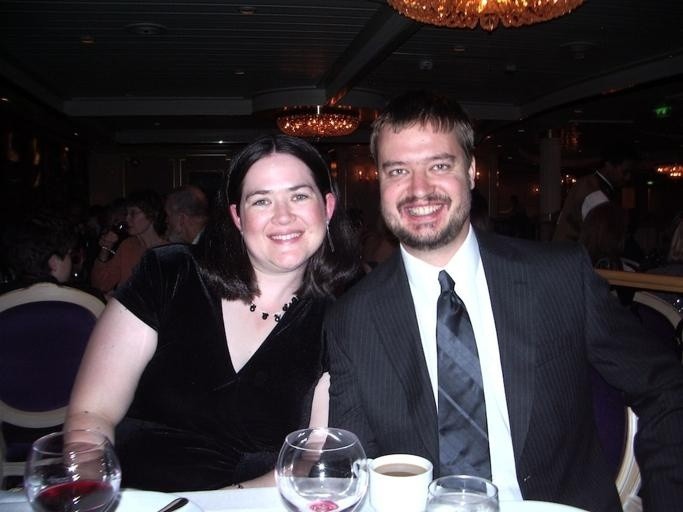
[386,0,586,32]
[275,105,362,138]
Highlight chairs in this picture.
[594,255,641,273]
[630,291,683,363]
[0,279,108,491]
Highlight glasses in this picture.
[123,209,144,217]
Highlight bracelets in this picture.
[230,482,244,489]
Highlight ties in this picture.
[435,268,495,487]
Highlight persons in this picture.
[0,206,109,488]
[550,138,638,269]
[63,134,361,494]
[0,184,214,303]
[336,206,395,300]
[469,190,494,235]
[319,86,682,512]
[642,216,682,318]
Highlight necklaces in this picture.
[248,288,299,323]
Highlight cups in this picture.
[369,453,433,512]
[23,429,122,512]
[426,475,500,512]
[275,427,369,512]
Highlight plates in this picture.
[119,490,205,512]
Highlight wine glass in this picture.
[102,219,128,255]
